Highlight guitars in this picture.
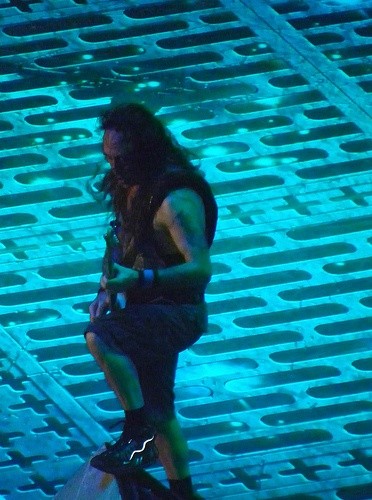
[102,227,127,316]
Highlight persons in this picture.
[83,100,219,500]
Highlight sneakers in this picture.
[90,426,159,472]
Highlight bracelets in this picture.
[142,268,154,293]
